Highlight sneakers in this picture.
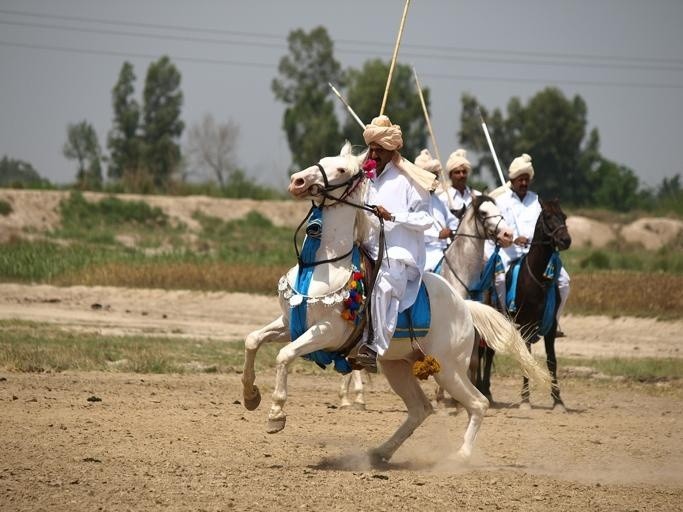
[347,351,379,366]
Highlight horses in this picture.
[339,187,513,411]
[241,140,559,469]
[477,190,571,413]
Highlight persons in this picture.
[413,150,466,273]
[350,115,434,373]
[485,153,571,338]
[435,147,496,318]
